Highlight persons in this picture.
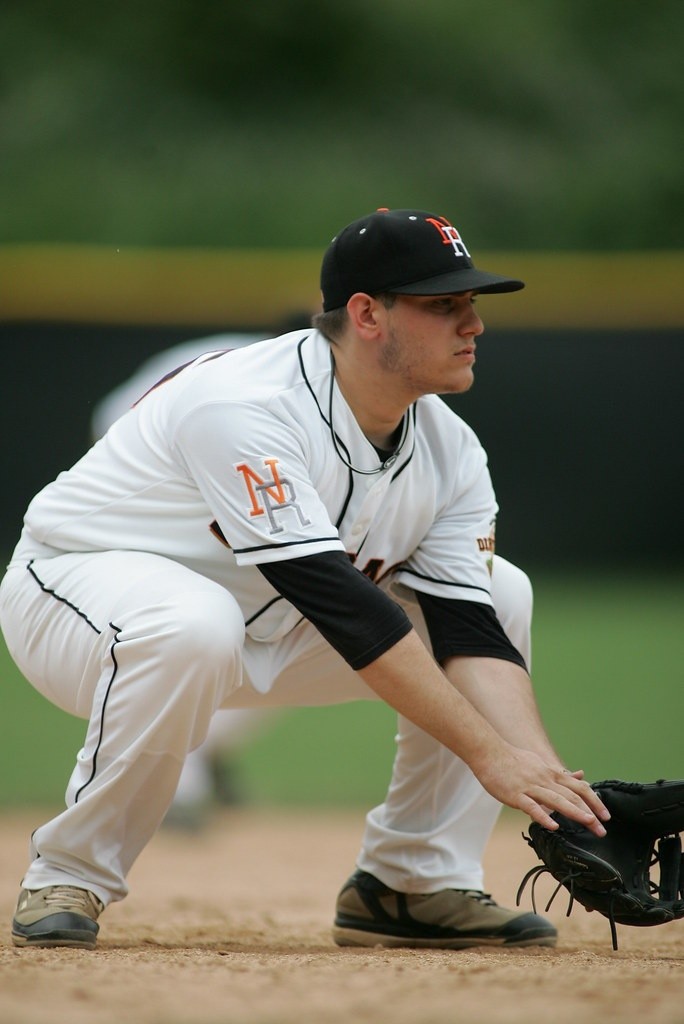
[0,206,612,951]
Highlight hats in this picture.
[319,207,525,313]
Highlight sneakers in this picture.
[11,885,104,951]
[331,869,558,950]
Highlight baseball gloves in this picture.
[511,779,684,949]
